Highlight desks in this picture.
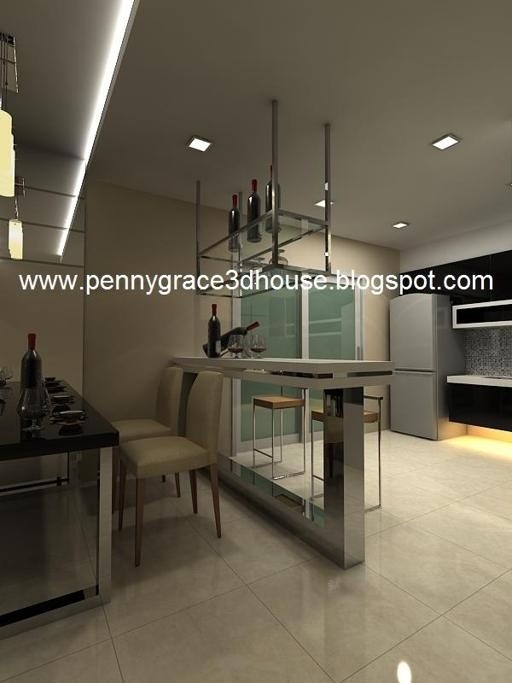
[172,352,396,573]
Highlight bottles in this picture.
[20,332,42,389]
[265,164,282,235]
[227,193,241,253]
[206,302,221,357]
[203,321,261,358]
[246,178,263,243]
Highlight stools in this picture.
[308,386,386,516]
[252,381,305,483]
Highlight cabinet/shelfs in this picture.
[186,95,335,299]
[446,375,512,431]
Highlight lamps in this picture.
[0,30,29,265]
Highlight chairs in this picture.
[105,358,191,533]
[115,365,231,569]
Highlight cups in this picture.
[44,374,85,425]
[59,426,87,436]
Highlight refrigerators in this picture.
[387,293,470,442]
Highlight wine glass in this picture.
[227,332,269,361]
[19,387,50,433]
